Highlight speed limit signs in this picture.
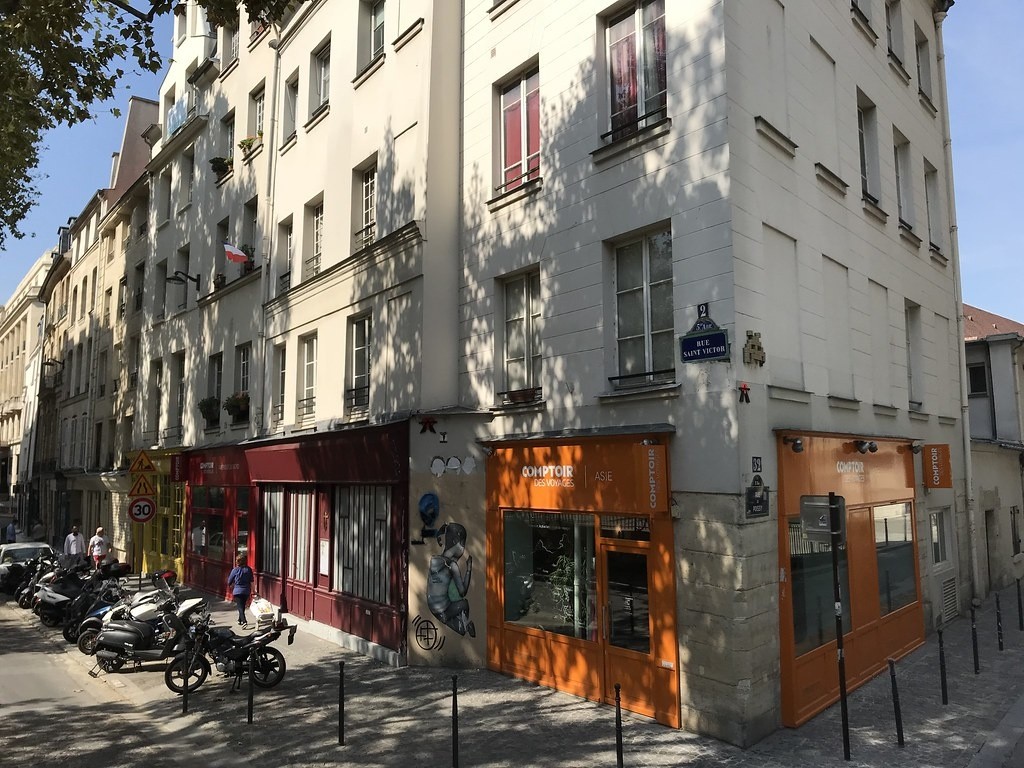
[128,498,157,523]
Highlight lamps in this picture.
[165,270,200,291]
[910,445,922,454]
[854,440,878,454]
[783,436,803,453]
[42,358,65,369]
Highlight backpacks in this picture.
[233,565,254,584]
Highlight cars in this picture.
[0,542,54,577]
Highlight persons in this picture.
[88,527,113,570]
[6,519,22,544]
[192,520,206,554]
[64,526,87,569]
[30,519,46,543]
[227,555,255,630]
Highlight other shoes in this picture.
[241,621,248,630]
[237,619,241,625]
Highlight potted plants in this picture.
[196,395,219,421]
[239,244,255,270]
[208,156,232,177]
[222,391,249,415]
[237,130,263,151]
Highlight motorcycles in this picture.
[163,612,291,695]
[6,546,215,675]
